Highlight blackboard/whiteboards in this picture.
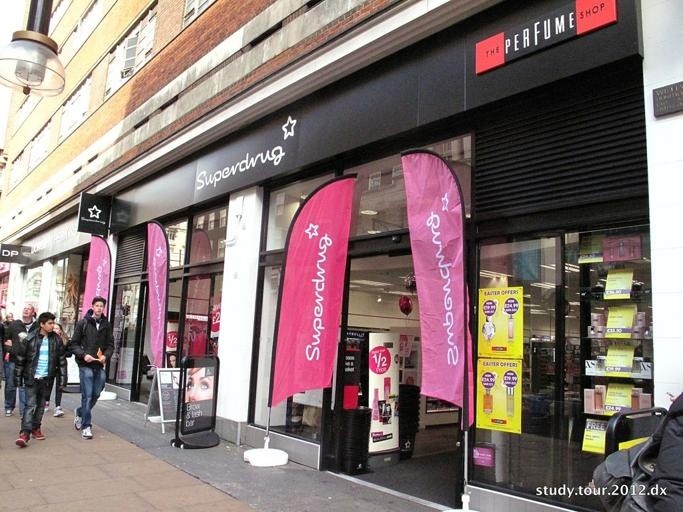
[160,371,184,420]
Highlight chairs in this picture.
[603,407,667,459]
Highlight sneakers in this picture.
[16,432,28,446]
[5,407,16,417]
[53,406,65,417]
[74,407,93,439]
[32,428,46,440]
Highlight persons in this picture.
[648,389,683,512]
[168,355,177,367]
[185,367,214,402]
[71,295,116,439]
[0,304,74,417]
[14,312,68,448]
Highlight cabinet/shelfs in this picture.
[390,326,461,431]
[570,226,654,456]
[527,336,555,393]
[562,335,581,403]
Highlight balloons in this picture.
[399,295,415,316]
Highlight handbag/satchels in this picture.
[592,437,656,511]
